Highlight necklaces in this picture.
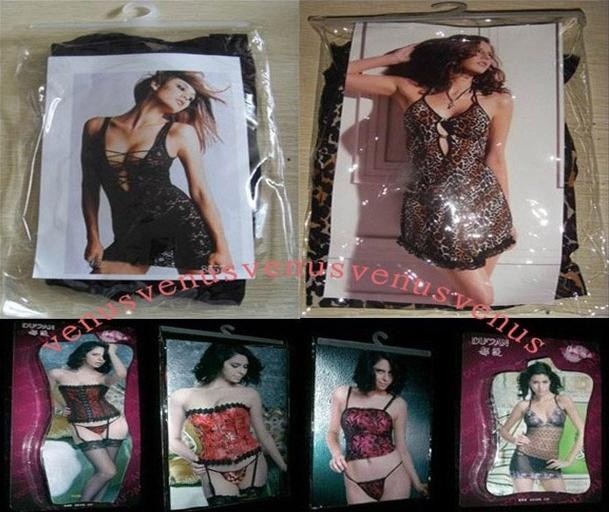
[445,86,472,108]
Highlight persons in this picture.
[167,339,287,507]
[325,350,430,505]
[499,362,585,493]
[81,70,235,274]
[343,34,514,305]
[47,340,129,504]
